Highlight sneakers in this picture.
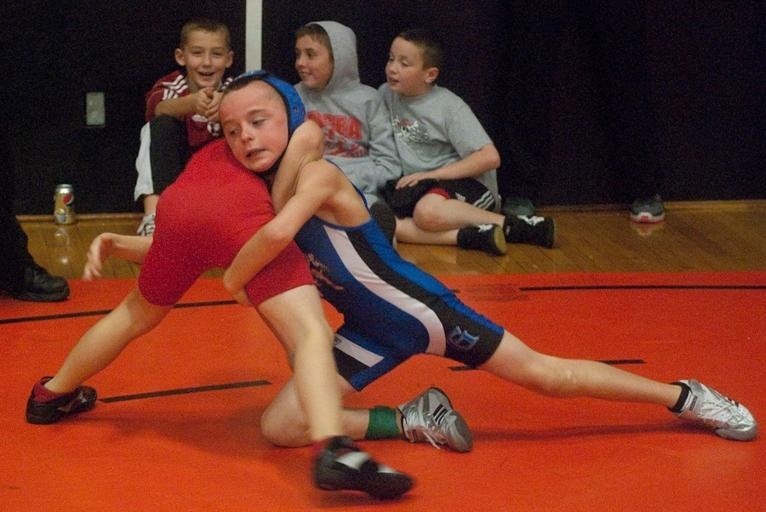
[503,214,557,249]
[501,192,535,218]
[316,438,413,495]
[672,378,757,441]
[629,194,667,225]
[137,211,157,236]
[3,260,70,303]
[397,387,473,453]
[455,222,508,256]
[26,377,97,424]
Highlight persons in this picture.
[290,19,404,250]
[26,116,415,499]
[130,16,238,235]
[0,0,69,301]
[375,28,553,254]
[84,68,759,451]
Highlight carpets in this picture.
[0,272,766,512]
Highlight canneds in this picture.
[53,183,76,225]
